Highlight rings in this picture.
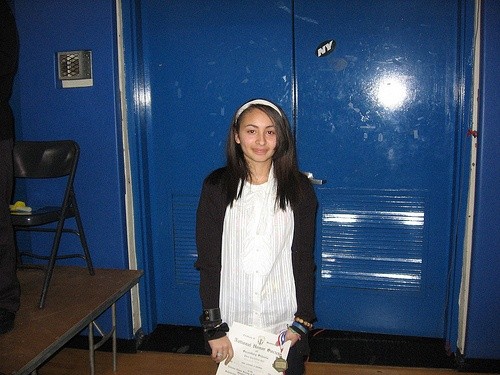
[217,353,223,356]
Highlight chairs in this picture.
[9,140,95,310]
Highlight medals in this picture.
[272,357,289,372]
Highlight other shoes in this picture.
[0,307,16,335]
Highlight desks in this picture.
[0,265,143,375]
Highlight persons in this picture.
[0,0,21,334]
[195,98,318,375]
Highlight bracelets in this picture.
[288,317,312,340]
